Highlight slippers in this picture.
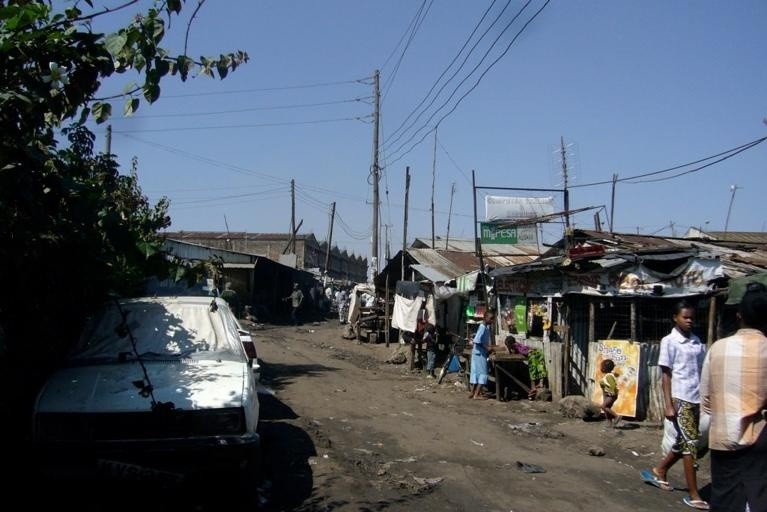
[638,468,674,492]
[682,493,711,510]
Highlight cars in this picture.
[31,295,262,473]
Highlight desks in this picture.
[465,350,529,401]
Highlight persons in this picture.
[424,323,442,380]
[467,312,497,399]
[649,300,709,504]
[307,278,386,325]
[504,337,546,398]
[699,286,767,510]
[597,358,619,428]
[281,279,304,323]
